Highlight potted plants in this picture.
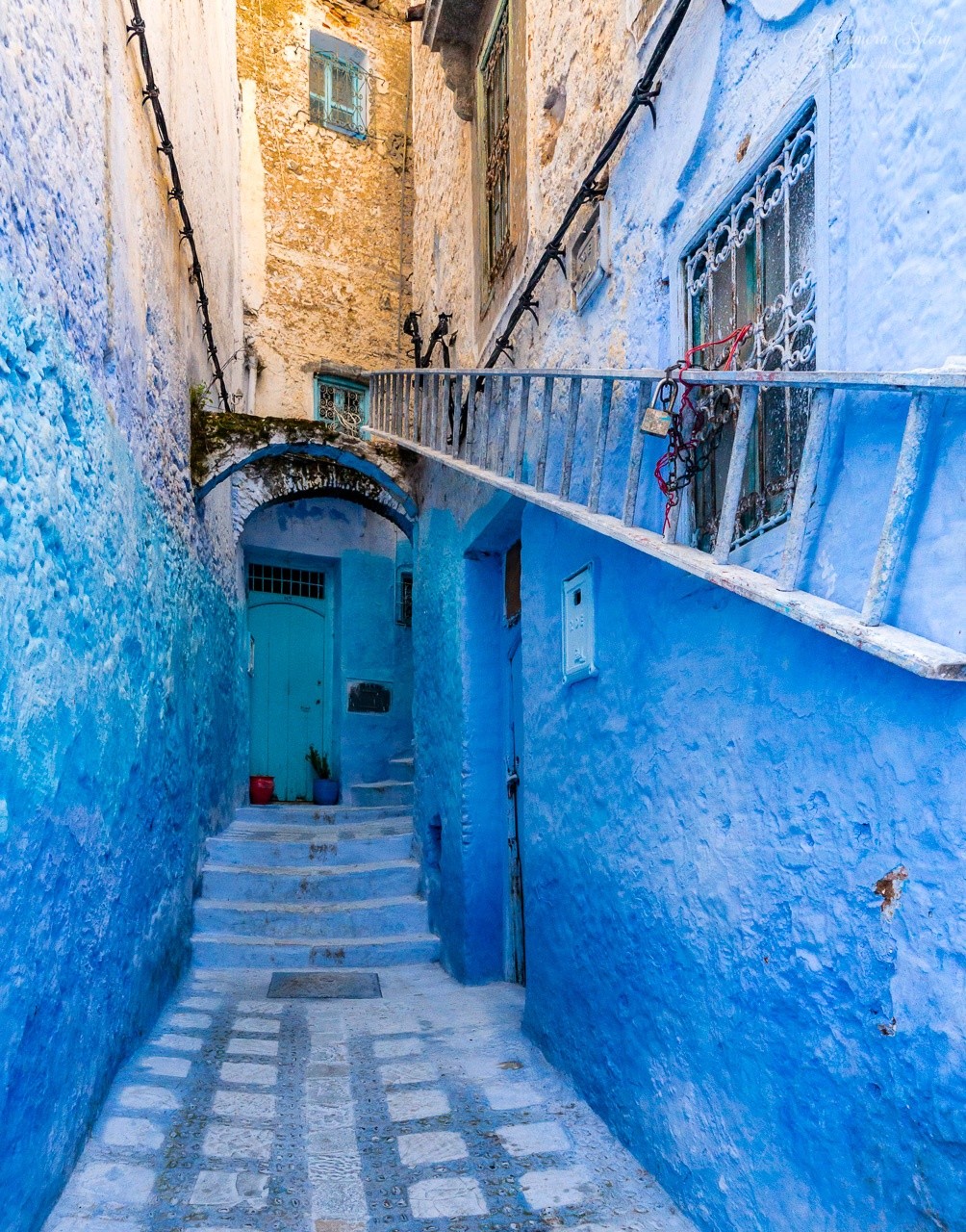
[305,746,338,805]
[250,773,276,804]
